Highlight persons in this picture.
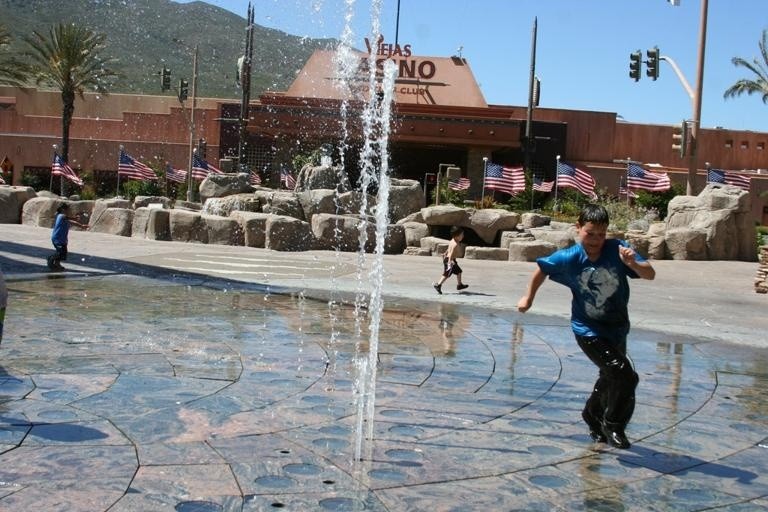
[431,303,462,358]
[518,204,656,450]
[0,271,9,345]
[433,224,469,295]
[45,202,90,271]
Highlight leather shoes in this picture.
[582,409,630,449]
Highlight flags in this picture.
[50,143,85,186]
[280,163,297,190]
[165,162,189,184]
[495,188,518,199]
[483,156,526,193]
[129,166,158,181]
[705,161,751,192]
[617,176,637,198]
[117,145,159,179]
[532,174,554,193]
[576,187,598,204]
[627,157,670,192]
[446,176,471,193]
[245,165,262,185]
[191,148,224,179]
[555,155,596,196]
[0,153,15,177]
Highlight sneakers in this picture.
[434,284,442,294]
[457,284,468,290]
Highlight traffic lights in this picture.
[672,123,687,159]
[647,45,659,80]
[630,50,642,81]
[427,175,436,184]
[178,79,188,102]
[161,68,171,92]
